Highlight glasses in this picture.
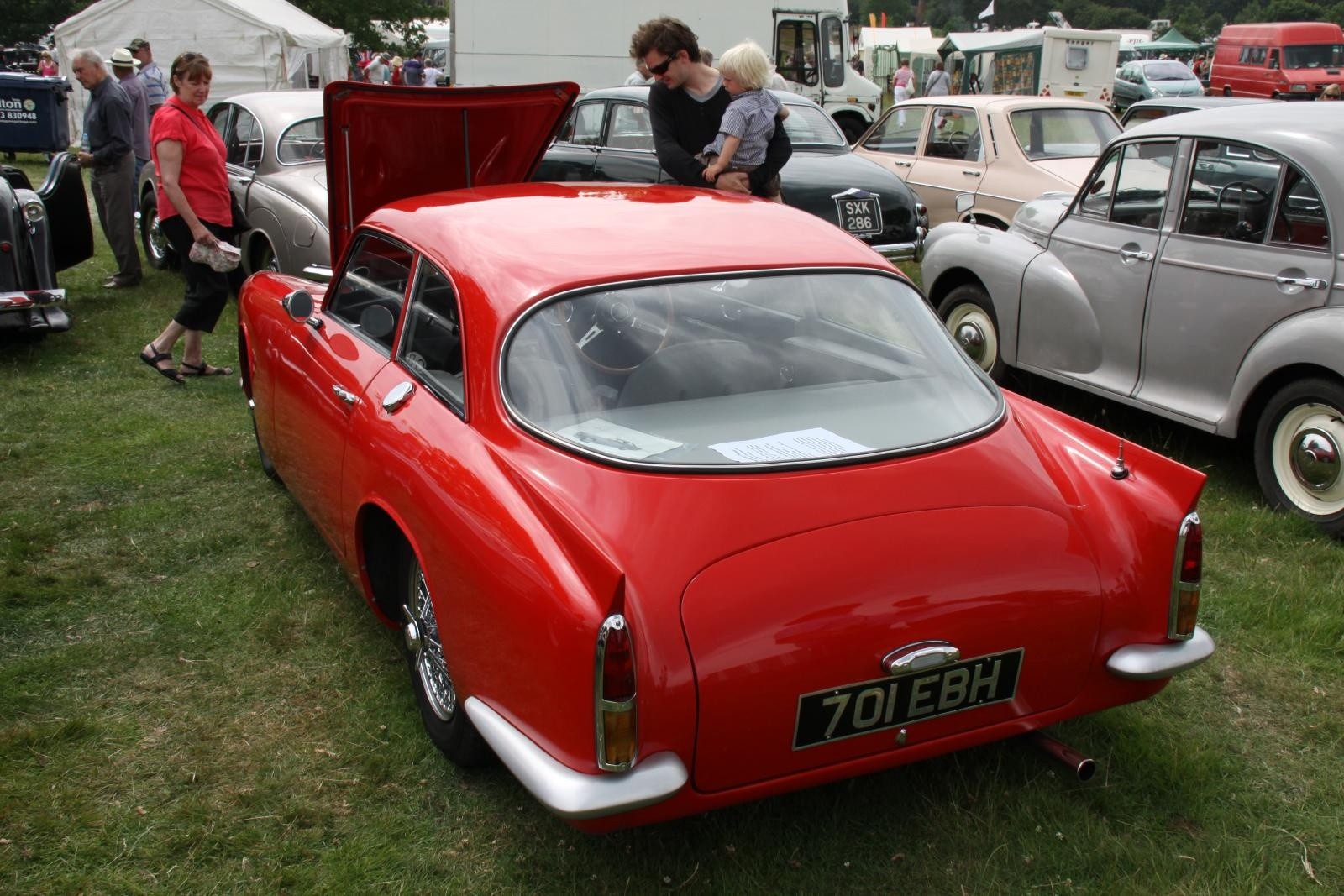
[175,52,195,65]
[648,52,675,74]
[1325,93,1339,100]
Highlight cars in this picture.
[852,17,1344,535]
[523,81,929,270]
[234,79,1222,837]
[849,93,1220,248]
[915,94,1343,538]
[137,86,334,286]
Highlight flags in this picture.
[978,0,994,19]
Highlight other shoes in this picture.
[103,281,139,292]
[105,275,116,280]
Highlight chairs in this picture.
[623,338,770,405]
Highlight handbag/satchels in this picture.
[230,190,252,235]
[906,68,915,95]
[189,232,241,273]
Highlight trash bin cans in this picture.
[0,73,70,153]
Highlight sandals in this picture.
[141,343,186,384]
[182,360,233,376]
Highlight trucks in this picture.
[449,0,888,148]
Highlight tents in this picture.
[1134,26,1207,60]
[55,0,348,149]
[859,25,1121,107]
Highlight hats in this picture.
[105,48,141,68]
[391,56,402,66]
[1198,55,1205,60]
[1158,54,1167,59]
[125,39,149,52]
[379,52,390,62]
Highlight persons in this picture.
[787,51,817,85]
[71,37,170,290]
[0,45,7,72]
[139,51,247,385]
[835,51,864,75]
[624,17,792,204]
[363,52,444,88]
[14,43,22,65]
[1153,51,1211,80]
[924,112,968,159]
[893,61,916,127]
[1316,84,1341,101]
[36,43,59,77]
[924,61,951,127]
